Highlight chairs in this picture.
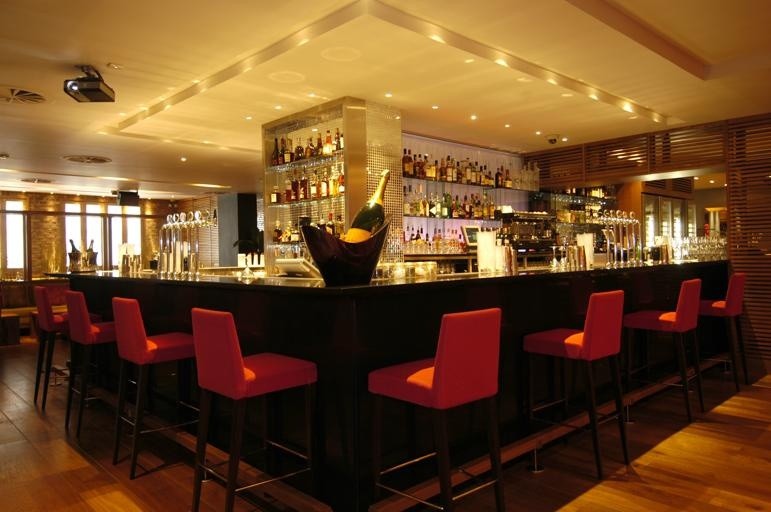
[188,305,318,511]
[621,279,707,425]
[110,295,195,481]
[363,305,507,512]
[518,289,632,483]
[58,289,111,442]
[30,283,100,415]
[698,271,751,394]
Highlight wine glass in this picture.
[653,236,728,263]
[549,246,567,268]
[241,256,254,277]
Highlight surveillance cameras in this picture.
[546,134,559,145]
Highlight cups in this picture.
[373,261,437,280]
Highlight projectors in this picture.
[63,63,116,103]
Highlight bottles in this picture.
[272,213,345,243]
[403,184,496,219]
[273,244,312,275]
[564,186,616,200]
[402,148,540,191]
[404,225,467,253]
[271,127,343,166]
[70,240,79,253]
[345,169,390,243]
[483,226,512,246]
[271,169,344,205]
[559,208,584,223]
[87,239,94,252]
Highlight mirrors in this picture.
[640,167,727,263]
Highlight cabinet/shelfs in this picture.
[398,171,547,261]
[261,96,347,275]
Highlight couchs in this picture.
[0,279,71,336]
